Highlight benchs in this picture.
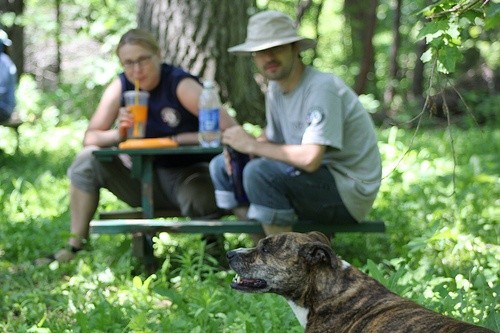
[89,219,386,281]
[99,210,175,258]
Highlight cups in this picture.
[124,90,149,138]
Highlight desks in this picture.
[91,135,254,278]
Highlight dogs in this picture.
[225,230,499,332]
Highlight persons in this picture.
[40,28,230,270]
[0,30,17,124]
[209,11,382,250]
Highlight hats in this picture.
[227,10,315,52]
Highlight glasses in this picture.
[122,56,154,68]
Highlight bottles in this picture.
[198,77,220,148]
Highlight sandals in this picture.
[26,245,92,267]
